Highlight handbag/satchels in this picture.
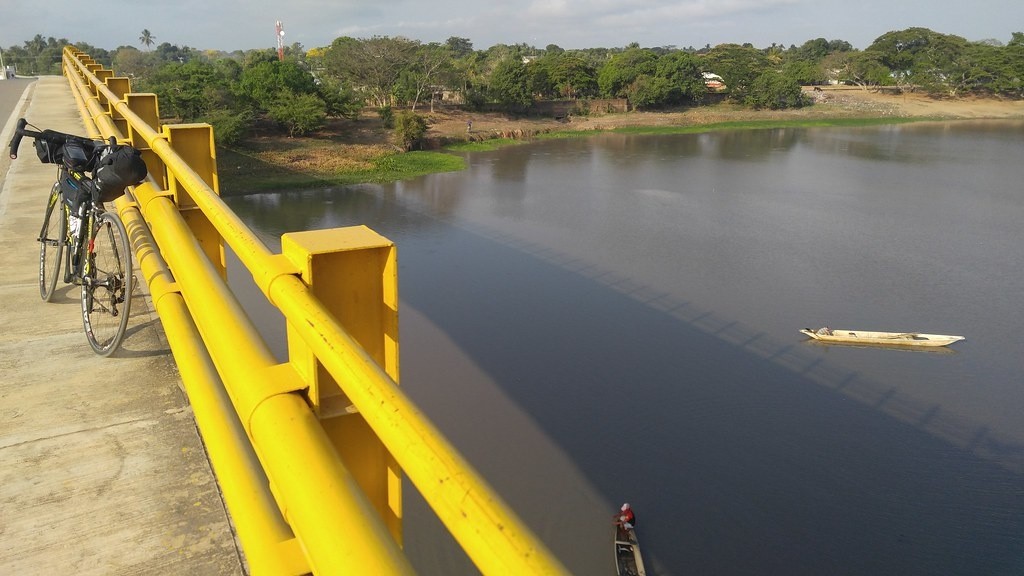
[87,145,147,202]
[34,128,97,172]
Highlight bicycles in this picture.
[7,118,142,357]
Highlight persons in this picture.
[612,503,636,534]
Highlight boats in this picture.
[614,520,646,576]
[799,329,965,346]
